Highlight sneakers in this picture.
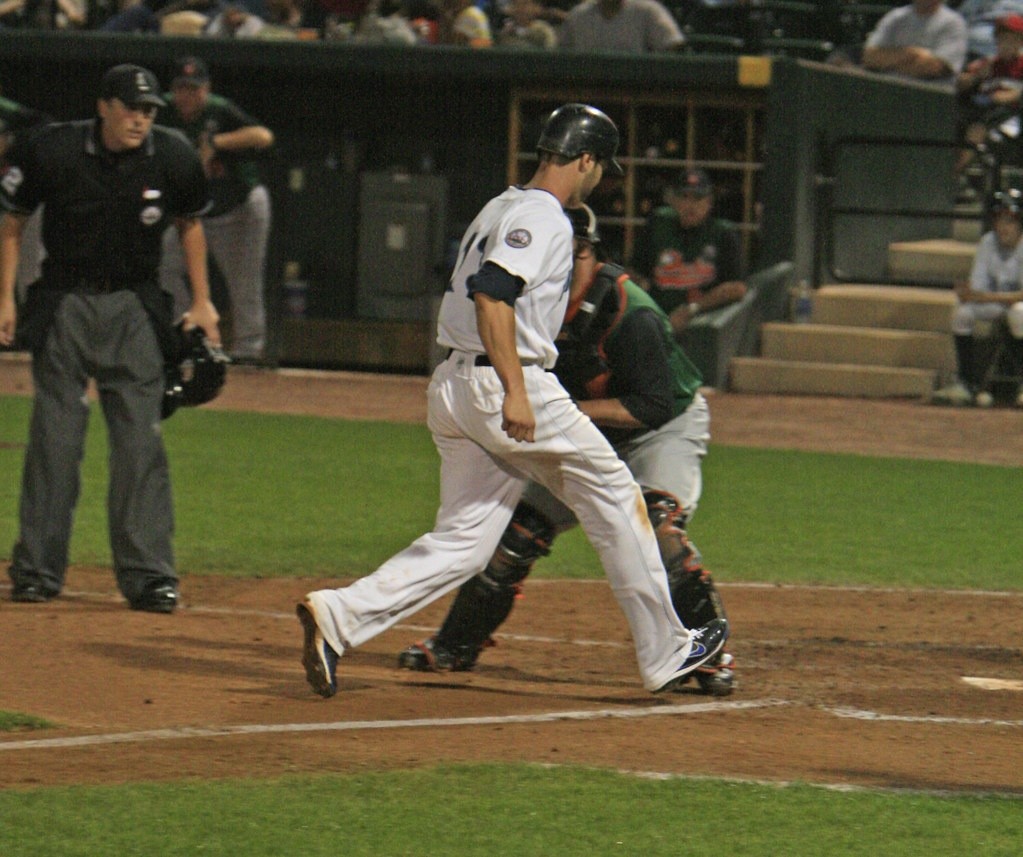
[674,666,736,692]
[651,616,728,695]
[401,642,478,672]
[296,600,337,698]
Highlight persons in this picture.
[160,58,275,363]
[559,0,684,53]
[863,0,1023,212]
[0,0,557,52]
[399,203,738,695]
[0,66,223,608]
[935,186,1023,404]
[297,102,729,698]
[0,100,44,295]
[628,170,747,332]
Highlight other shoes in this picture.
[930,385,976,405]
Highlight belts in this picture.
[445,348,492,367]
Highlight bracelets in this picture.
[690,303,700,314]
[206,136,218,151]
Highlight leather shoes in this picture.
[12,581,49,602]
[132,585,179,612]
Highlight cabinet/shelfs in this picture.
[507,85,764,283]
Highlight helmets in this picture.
[563,204,601,245]
[537,102,623,175]
[988,186,1022,221]
[159,323,225,419]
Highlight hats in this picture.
[175,56,209,87]
[100,65,166,106]
[676,169,711,195]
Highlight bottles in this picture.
[795,280,811,324]
